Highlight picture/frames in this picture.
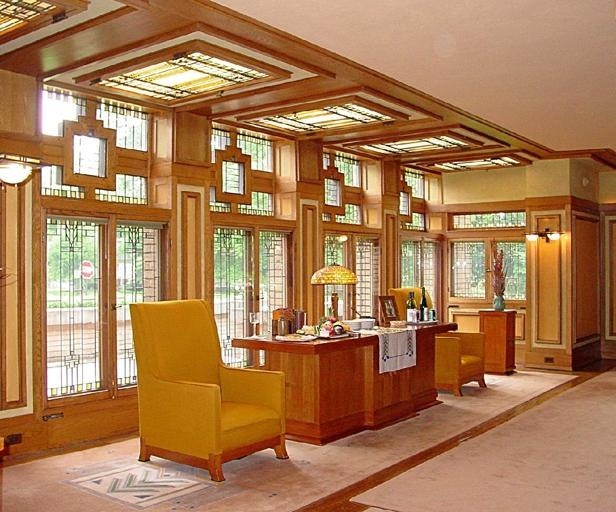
[380,296,400,322]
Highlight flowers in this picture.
[493,250,507,296]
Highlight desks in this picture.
[231,324,460,446]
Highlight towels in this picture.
[366,327,418,374]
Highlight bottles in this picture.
[406,287,429,323]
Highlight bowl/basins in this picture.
[341,319,375,331]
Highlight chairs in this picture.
[129,298,290,481]
[389,287,487,397]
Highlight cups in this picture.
[429,309,436,321]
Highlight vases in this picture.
[493,296,505,310]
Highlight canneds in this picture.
[429,310,435,322]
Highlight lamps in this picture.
[524,231,563,243]
[0,159,34,190]
[311,263,358,320]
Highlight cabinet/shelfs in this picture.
[475,309,517,373]
